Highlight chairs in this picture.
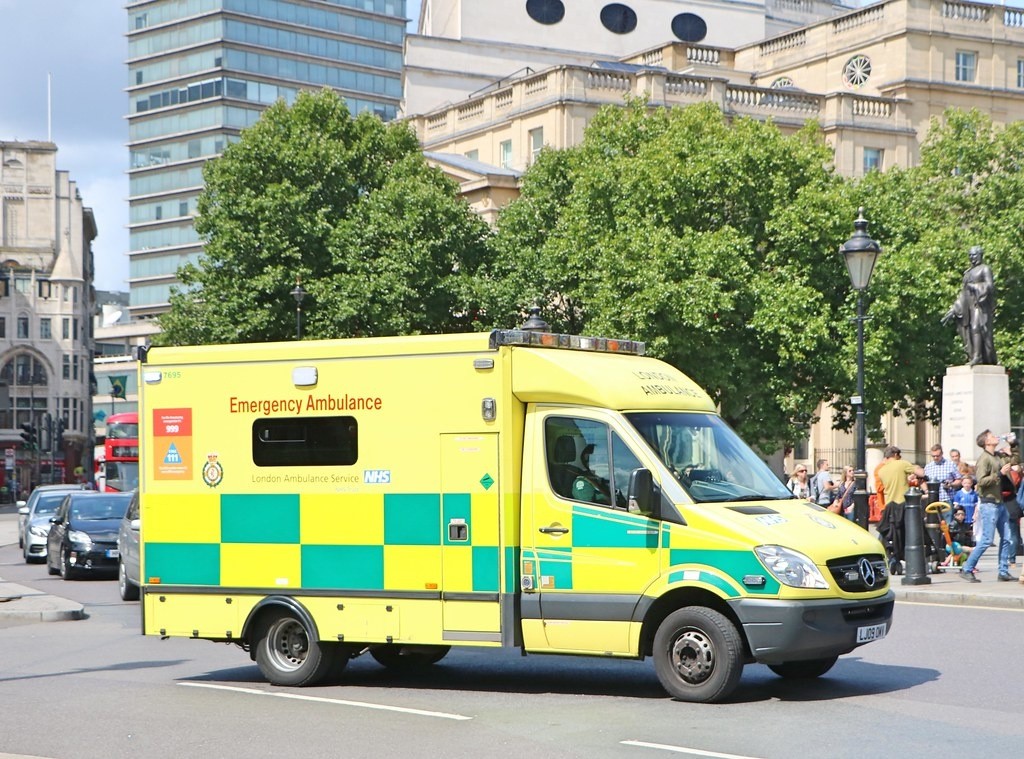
[552,435,584,498]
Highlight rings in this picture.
[1006,467,1008,469]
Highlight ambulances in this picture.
[128,325,897,704]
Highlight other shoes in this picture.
[1010,562,1017,567]
[890,562,896,575]
[959,568,982,583]
[939,561,947,566]
[998,571,1019,582]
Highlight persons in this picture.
[669,430,1024,584]
[939,247,996,366]
[571,444,626,508]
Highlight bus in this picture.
[93,413,138,492]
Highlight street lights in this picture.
[289,282,309,341]
[836,203,881,530]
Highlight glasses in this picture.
[931,453,942,457]
[846,469,854,472]
[798,469,807,473]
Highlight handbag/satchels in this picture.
[827,499,843,514]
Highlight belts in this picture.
[981,496,1001,504]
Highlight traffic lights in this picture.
[19,421,31,443]
[56,418,66,447]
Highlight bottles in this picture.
[999,432,1016,440]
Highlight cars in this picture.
[45,491,135,581]
[19,489,83,563]
[14,483,96,549]
[115,487,141,602]
